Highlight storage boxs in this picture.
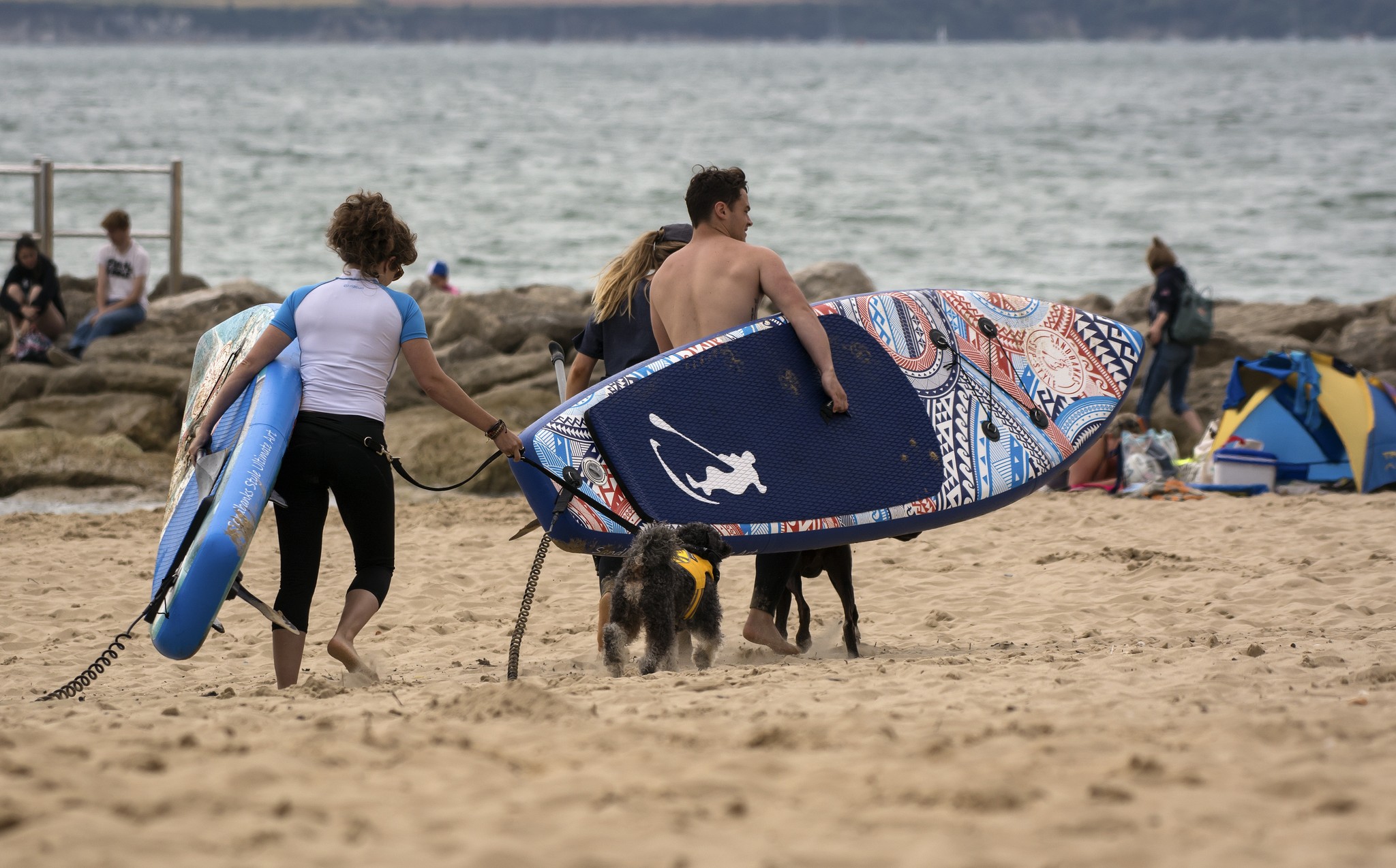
[1215,449,1277,490]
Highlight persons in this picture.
[650,164,848,655]
[48,210,152,365]
[1136,237,1206,445]
[429,260,460,294]
[185,186,524,692]
[0,232,69,357]
[1045,413,1143,491]
[567,223,694,666]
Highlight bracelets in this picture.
[485,418,507,443]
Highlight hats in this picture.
[428,260,448,278]
[659,223,692,244]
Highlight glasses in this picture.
[392,260,404,282]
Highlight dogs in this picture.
[600,519,728,681]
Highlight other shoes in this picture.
[47,345,80,368]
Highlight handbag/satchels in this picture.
[18,325,53,362]
[1173,278,1213,344]
[1121,427,1181,492]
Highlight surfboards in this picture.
[506,287,1148,557]
[150,300,302,662]
